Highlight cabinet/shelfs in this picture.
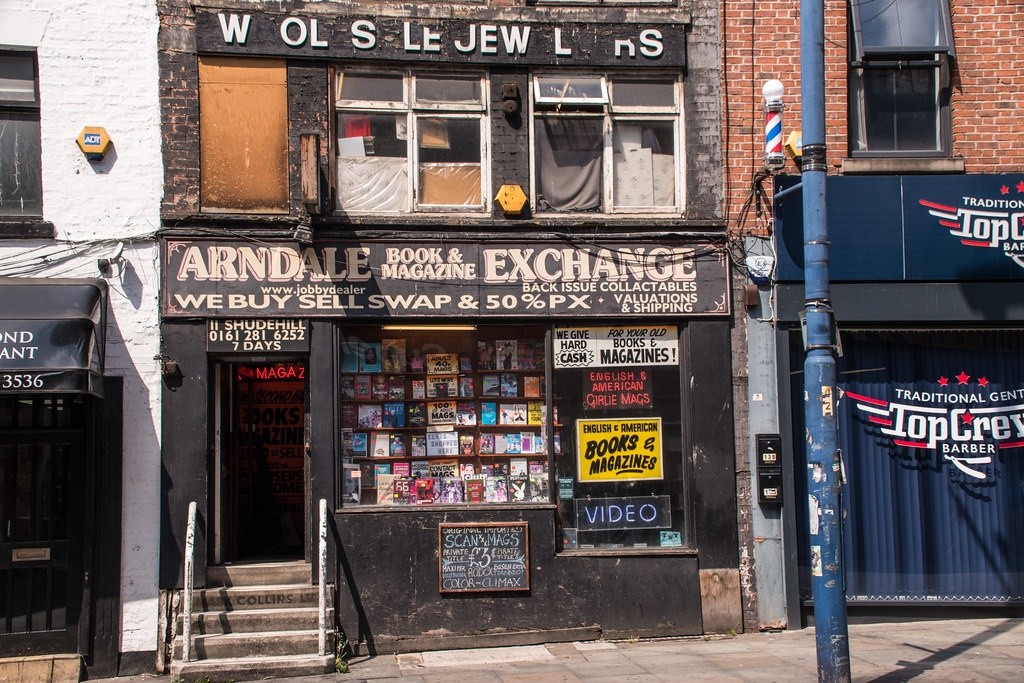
[339,370,550,507]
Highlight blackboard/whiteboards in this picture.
[438,521,531,594]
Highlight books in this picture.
[341,340,561,504]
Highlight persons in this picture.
[464,464,474,475]
[391,437,405,454]
[344,468,358,503]
[497,480,506,499]
[515,408,525,421]
[373,375,388,391]
[388,409,398,427]
[502,410,508,423]
[413,438,425,453]
[500,342,515,371]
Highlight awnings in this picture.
[0,278,107,402]
